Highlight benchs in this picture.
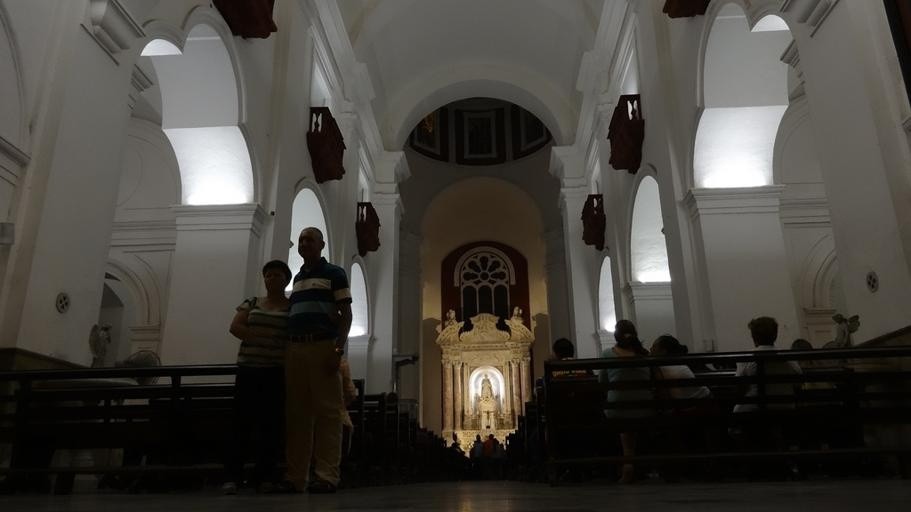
[505,345,911,487]
[1,364,447,490]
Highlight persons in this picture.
[481,374,493,398]
[825,313,849,348]
[386,391,399,413]
[734,317,837,411]
[553,320,710,456]
[471,434,504,479]
[223,227,354,493]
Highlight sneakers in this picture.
[257,480,300,494]
[222,482,236,493]
[308,477,337,493]
